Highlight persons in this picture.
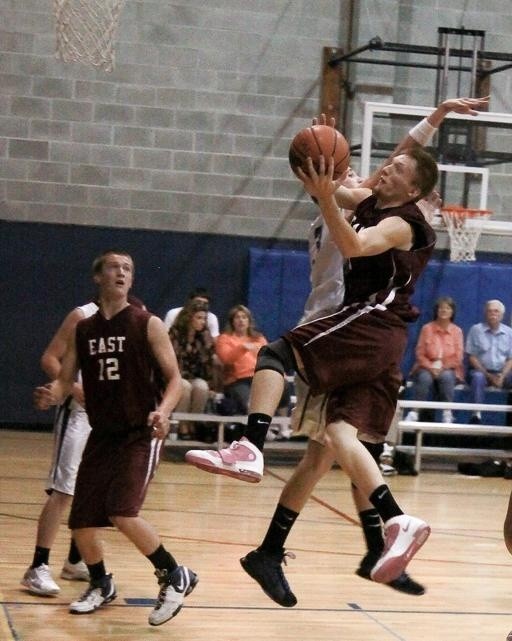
[407,296,512,423]
[239,95,493,609]
[33,252,198,625]
[19,258,113,596]
[163,292,294,443]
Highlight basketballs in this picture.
[289,125,350,183]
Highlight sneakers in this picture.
[148,566,198,625]
[20,557,117,614]
[185,437,263,482]
[406,409,480,423]
[356,514,431,593]
[240,547,297,607]
